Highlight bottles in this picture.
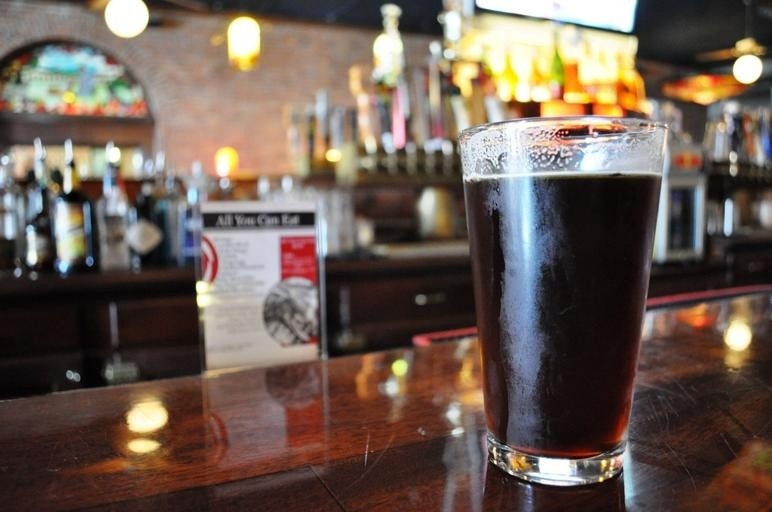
[0,138,234,276]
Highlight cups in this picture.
[458,117,668,488]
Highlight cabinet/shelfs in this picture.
[300,173,478,362]
[705,161,772,289]
[0,178,202,398]
